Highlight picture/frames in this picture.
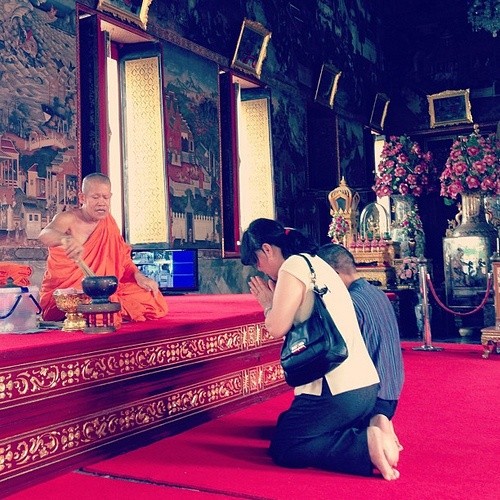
[231,19,272,79]
[314,63,342,109]
[369,93,391,131]
[95,0,153,30]
[426,88,474,129]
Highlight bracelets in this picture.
[264,306,272,317]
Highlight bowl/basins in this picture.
[81,276,118,304]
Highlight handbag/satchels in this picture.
[280,252,348,387]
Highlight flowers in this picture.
[394,211,423,235]
[399,258,418,281]
[441,127,500,202]
[372,133,432,196]
[327,217,351,246]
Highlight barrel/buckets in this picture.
[0,285,42,334]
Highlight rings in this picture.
[256,291,259,295]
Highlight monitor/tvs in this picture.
[129,249,200,295]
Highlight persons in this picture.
[239,218,404,481]
[38,172,159,321]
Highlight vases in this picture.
[446,192,497,295]
[391,194,426,257]
[408,236,416,256]
